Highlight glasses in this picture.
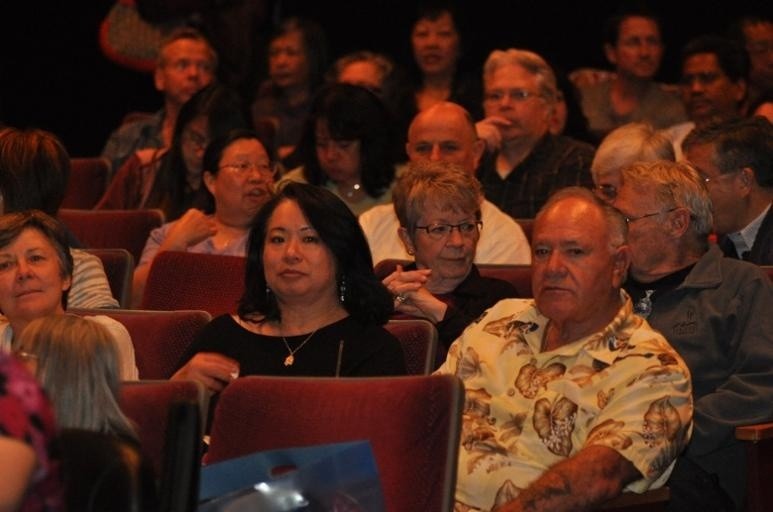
[413,220,484,238]
[593,183,617,201]
[623,206,677,226]
[211,158,270,176]
[697,170,742,185]
[180,131,210,151]
[487,88,543,102]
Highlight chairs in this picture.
[59,155,112,210]
[382,318,439,376]
[63,307,212,381]
[375,257,533,299]
[76,245,135,310]
[139,248,248,319]
[200,373,466,512]
[119,378,209,512]
[54,207,166,265]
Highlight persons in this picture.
[276,83,403,215]
[323,47,395,86]
[94,28,220,175]
[91,82,254,211]
[254,15,326,143]
[429,187,694,512]
[473,48,596,220]
[0,209,139,385]
[373,161,522,358]
[162,181,408,466]
[592,120,679,208]
[358,100,532,266]
[0,127,75,212]
[609,159,772,512]
[681,108,772,270]
[578,8,684,136]
[0,344,57,512]
[740,14,773,113]
[13,317,144,512]
[656,27,754,163]
[397,2,487,144]
[129,131,276,313]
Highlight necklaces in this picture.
[260,298,341,369]
[336,175,365,202]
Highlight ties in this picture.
[632,289,655,319]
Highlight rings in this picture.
[393,292,408,306]
